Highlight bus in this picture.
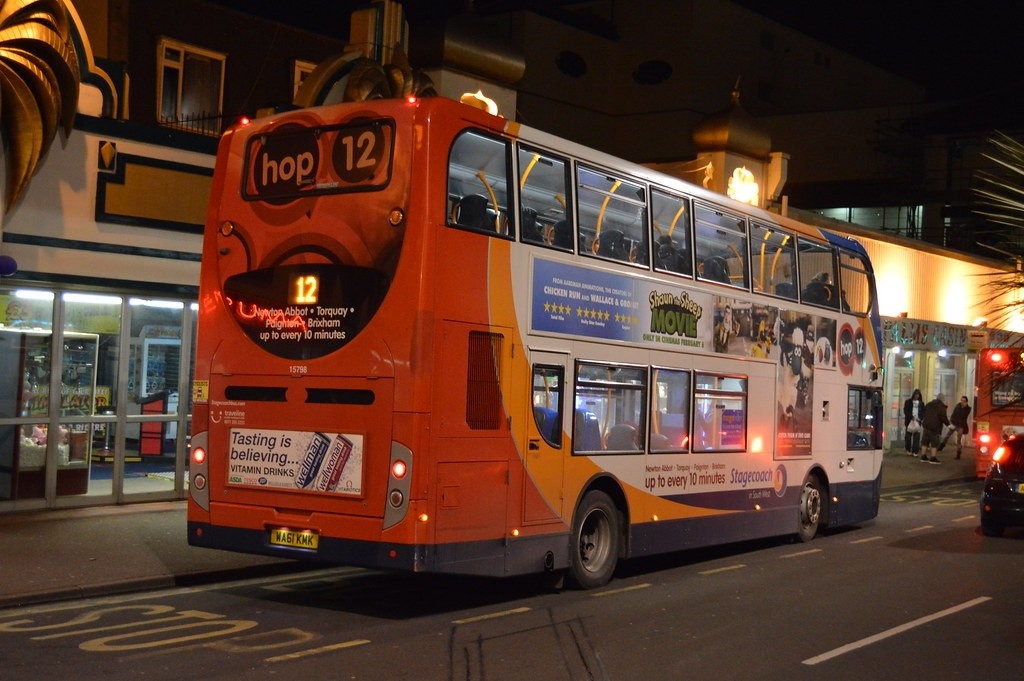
[975,347,1024,480]
[184,94,887,591]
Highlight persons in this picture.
[657,235,686,259]
[904,389,925,457]
[937,396,971,460]
[920,393,955,464]
[812,272,850,311]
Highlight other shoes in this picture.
[913,452,918,456]
[955,454,961,459]
[907,449,912,456]
[937,444,943,451]
[929,457,941,464]
[921,455,929,462]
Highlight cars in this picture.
[979,432,1024,535]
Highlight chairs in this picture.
[536,404,696,451]
[450,193,852,315]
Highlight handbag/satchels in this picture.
[962,424,969,434]
[907,420,921,433]
